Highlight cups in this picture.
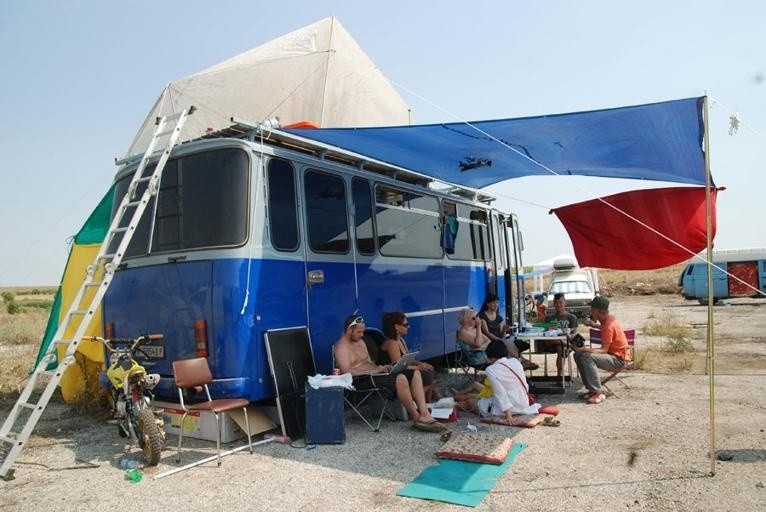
[333,369,341,376]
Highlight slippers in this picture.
[414,419,447,432]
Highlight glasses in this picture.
[348,316,365,326]
[400,323,408,328]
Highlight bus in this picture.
[98,122,529,401]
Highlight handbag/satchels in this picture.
[529,393,536,406]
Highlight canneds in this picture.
[332,368,340,375]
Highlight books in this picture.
[567,331,585,347]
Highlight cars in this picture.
[543,273,596,320]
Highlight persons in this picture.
[571,296,630,404]
[456,307,539,371]
[444,378,494,398]
[334,314,448,432]
[379,312,436,411]
[544,293,578,386]
[458,340,542,428]
[475,294,505,338]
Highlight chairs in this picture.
[168,357,255,470]
[331,345,397,431]
[361,327,387,365]
[586,326,636,401]
[452,331,491,390]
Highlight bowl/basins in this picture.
[523,323,561,338]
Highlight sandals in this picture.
[522,359,539,372]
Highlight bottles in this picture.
[125,468,143,483]
[118,458,139,471]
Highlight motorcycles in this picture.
[80,329,167,467]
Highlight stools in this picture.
[541,347,575,386]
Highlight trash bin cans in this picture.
[305,377,345,444]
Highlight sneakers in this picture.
[583,390,606,404]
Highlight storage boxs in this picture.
[153,399,277,445]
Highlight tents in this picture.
[27,183,115,405]
[109,15,413,170]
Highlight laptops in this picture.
[371,351,419,376]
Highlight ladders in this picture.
[0,106,198,477]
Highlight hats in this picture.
[587,297,609,310]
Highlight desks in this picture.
[520,334,576,393]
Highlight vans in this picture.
[678,248,766,305]
